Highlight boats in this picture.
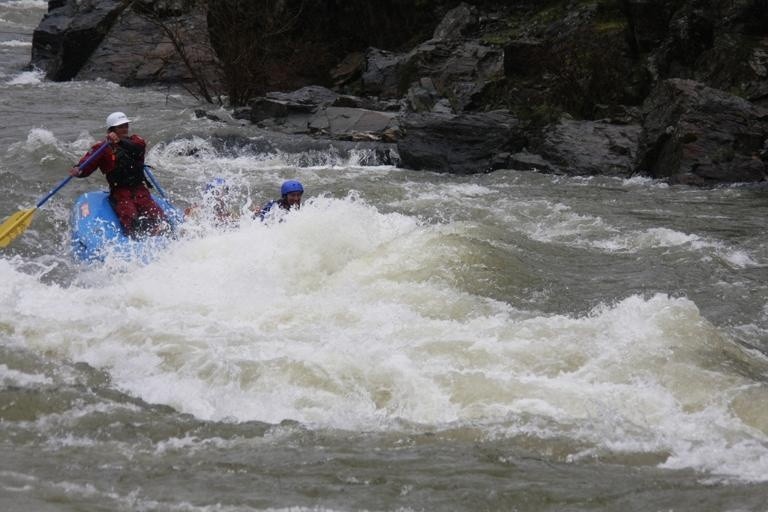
[71,189,184,267]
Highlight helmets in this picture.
[280,178,305,198]
[105,109,133,130]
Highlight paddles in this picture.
[0,134,119,248]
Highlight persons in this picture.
[73,111,170,242]
[256,179,306,220]
[184,177,261,229]
